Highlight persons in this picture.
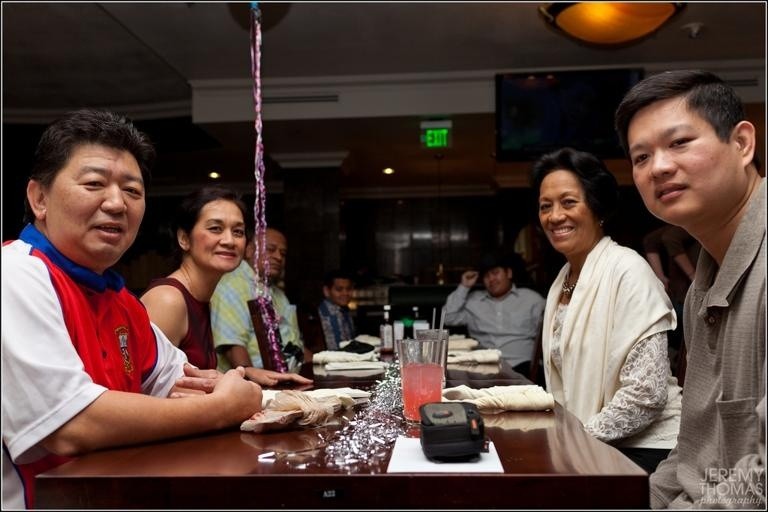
[615,68,767,509]
[528,147,683,476]
[442,255,546,381]
[137,183,248,369]
[210,228,305,375]
[641,223,702,293]
[305,271,357,353]
[1,107,263,509]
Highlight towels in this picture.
[312,333,501,370]
[241,390,354,432]
[441,384,555,415]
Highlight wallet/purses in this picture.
[418,402,490,464]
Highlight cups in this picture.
[416,328,450,386]
[397,338,446,428]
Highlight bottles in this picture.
[380,311,394,352]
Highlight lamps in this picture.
[540,1,687,45]
